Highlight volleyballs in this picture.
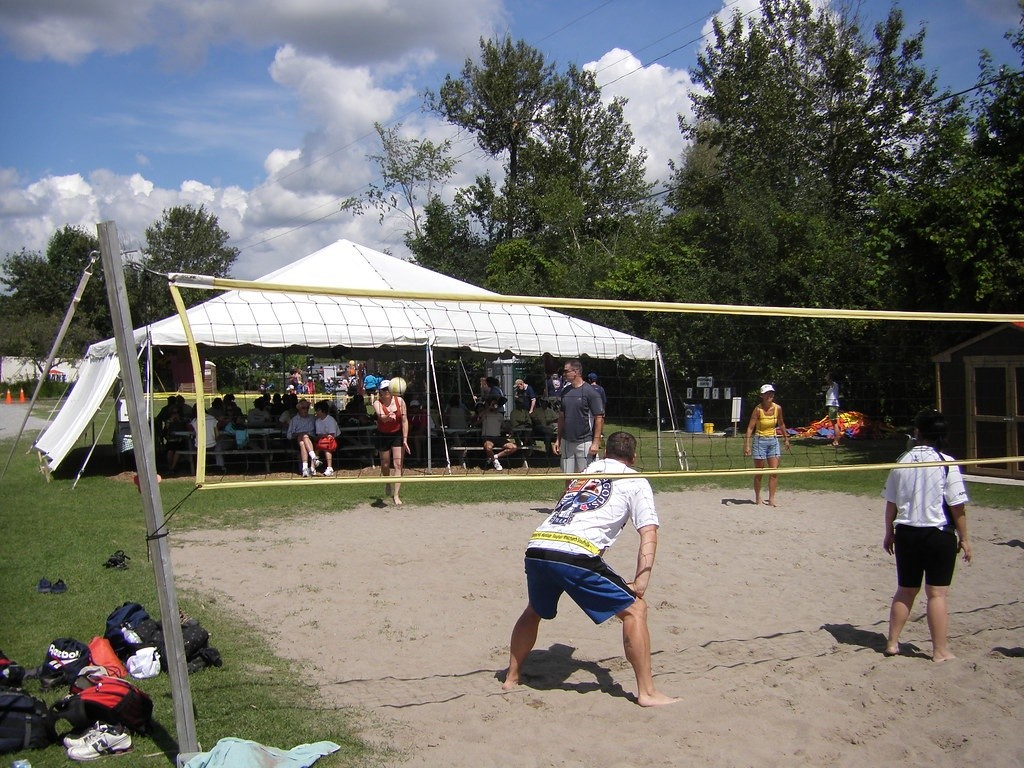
[388,377,407,396]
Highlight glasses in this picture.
[302,406,310,409]
[563,369,579,373]
[382,387,389,391]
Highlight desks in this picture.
[169,424,381,476]
[444,426,604,469]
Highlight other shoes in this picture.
[323,467,334,476]
[52,579,68,593]
[38,577,51,593]
[313,456,323,467]
[302,467,317,477]
[485,457,503,471]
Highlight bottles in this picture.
[121,627,143,643]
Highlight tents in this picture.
[0,240,691,490]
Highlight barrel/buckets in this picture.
[684,404,703,432]
[704,423,714,434]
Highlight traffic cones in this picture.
[17,387,27,404]
[4,389,14,404]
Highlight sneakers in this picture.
[63,720,133,761]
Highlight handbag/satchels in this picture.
[497,396,508,407]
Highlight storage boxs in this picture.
[116,395,151,423]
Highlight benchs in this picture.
[175,442,375,462]
[450,445,603,463]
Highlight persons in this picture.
[404,368,608,470]
[310,401,341,478]
[260,363,386,406]
[155,392,367,464]
[502,431,683,706]
[287,399,321,478]
[880,411,972,662]
[743,384,793,507]
[554,359,606,492]
[826,372,842,446]
[373,379,410,505]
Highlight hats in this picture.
[410,400,420,409]
[513,379,523,388]
[760,384,775,393]
[588,373,597,381]
[296,398,311,409]
[379,380,390,389]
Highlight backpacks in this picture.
[103,550,130,571]
[0,601,222,756]
[97,706,98,706]
